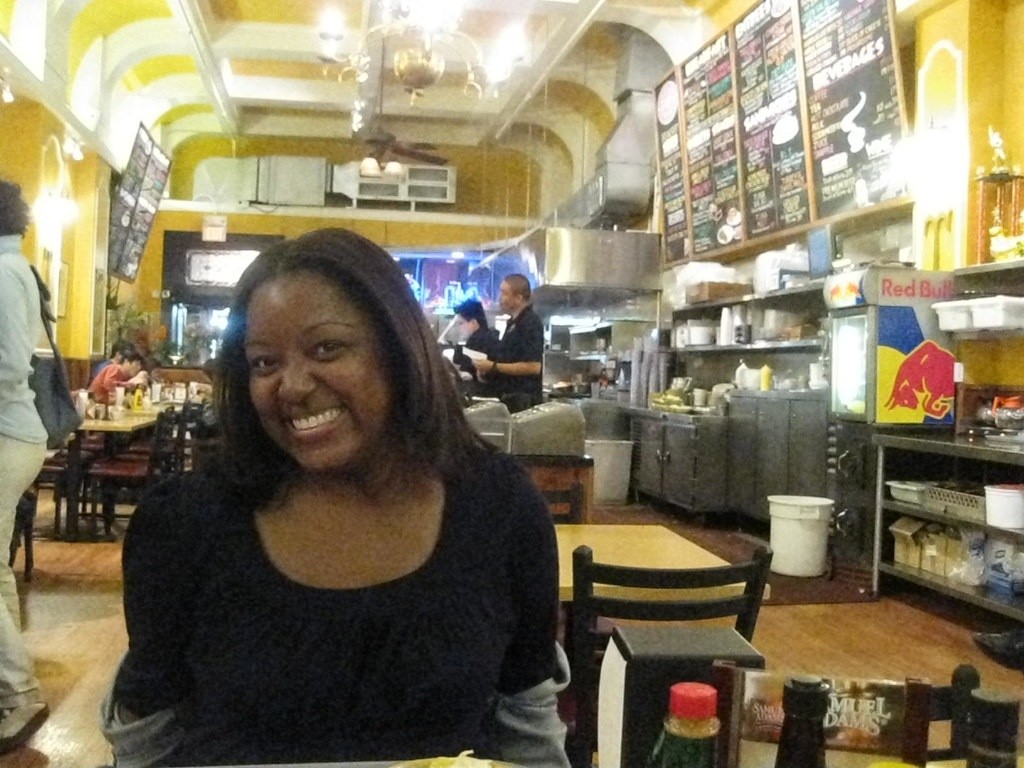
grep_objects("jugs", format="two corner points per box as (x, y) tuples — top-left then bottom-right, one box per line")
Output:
(991, 395), (1024, 430)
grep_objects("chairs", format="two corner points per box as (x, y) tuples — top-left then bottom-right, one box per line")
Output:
(566, 539), (774, 709)
(33, 381), (212, 544)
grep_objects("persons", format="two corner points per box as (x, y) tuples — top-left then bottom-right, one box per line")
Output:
(98, 227), (573, 768)
(454, 300), (501, 377)
(471, 273), (545, 413)
(1, 178), (53, 752)
(89, 339), (147, 406)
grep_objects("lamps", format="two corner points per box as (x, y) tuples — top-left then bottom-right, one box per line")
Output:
(357, 131), (405, 178)
(314, 1), (527, 111)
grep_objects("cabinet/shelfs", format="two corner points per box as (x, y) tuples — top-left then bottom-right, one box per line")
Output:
(625, 409), (725, 514)
(869, 432), (1024, 635)
(666, 275), (829, 355)
(947, 260), (1024, 340)
(728, 391), (828, 525)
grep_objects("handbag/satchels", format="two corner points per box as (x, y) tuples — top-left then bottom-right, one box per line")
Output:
(30, 264), (83, 451)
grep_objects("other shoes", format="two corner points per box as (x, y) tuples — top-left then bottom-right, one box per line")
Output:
(0, 701), (49, 752)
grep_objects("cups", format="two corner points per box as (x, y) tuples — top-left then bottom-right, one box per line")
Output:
(675, 304), (802, 346)
(629, 335), (668, 408)
(691, 388), (708, 405)
(591, 382), (599, 399)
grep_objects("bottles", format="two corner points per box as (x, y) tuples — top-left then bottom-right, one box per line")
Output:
(646, 681), (720, 768)
(775, 673), (830, 768)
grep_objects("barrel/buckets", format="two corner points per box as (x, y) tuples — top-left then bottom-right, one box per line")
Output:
(583, 440), (634, 506)
(766, 494), (835, 577)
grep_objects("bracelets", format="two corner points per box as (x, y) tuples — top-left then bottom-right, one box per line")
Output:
(490, 361), (497, 374)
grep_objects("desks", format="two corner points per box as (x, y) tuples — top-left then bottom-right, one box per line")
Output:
(550, 523), (771, 631)
